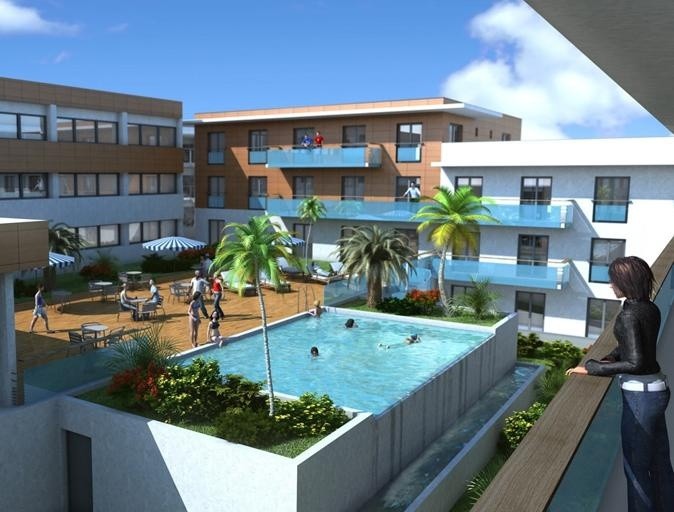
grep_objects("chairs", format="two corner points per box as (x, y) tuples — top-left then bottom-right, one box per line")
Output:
(115, 270), (211, 323)
(220, 256), (345, 299)
(88, 280), (104, 303)
(69, 326), (125, 352)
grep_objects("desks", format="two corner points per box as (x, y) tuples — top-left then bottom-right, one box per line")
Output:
(81, 322), (109, 348)
(94, 282), (112, 301)
(52, 291), (72, 313)
